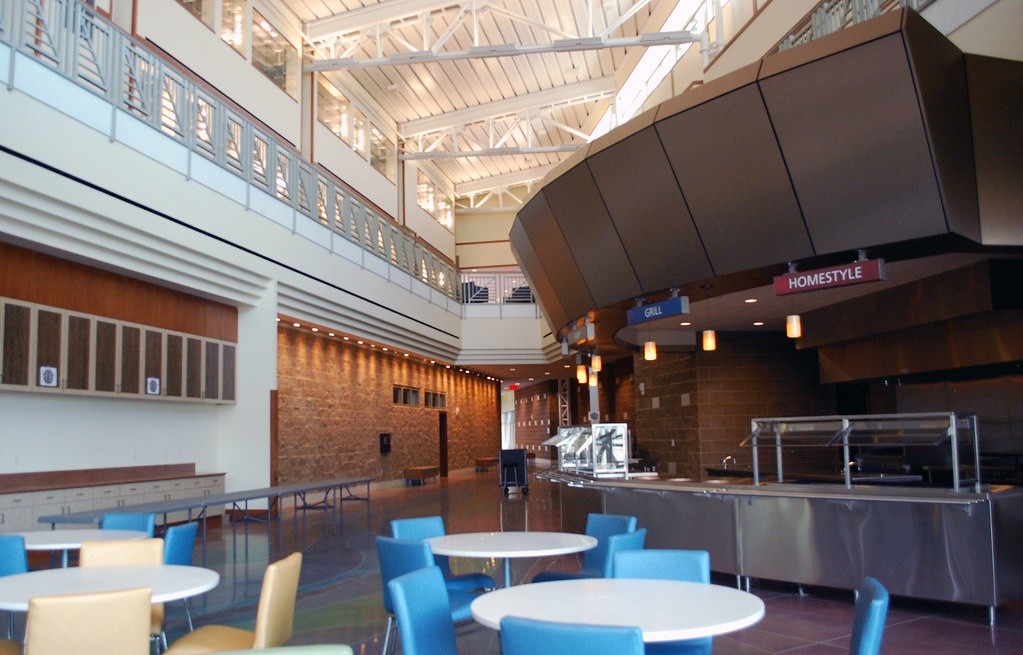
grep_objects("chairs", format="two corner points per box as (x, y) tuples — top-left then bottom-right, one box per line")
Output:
(849, 574), (888, 655)
(0, 511), (713, 655)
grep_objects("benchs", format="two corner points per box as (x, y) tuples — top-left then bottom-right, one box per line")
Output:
(404, 466), (439, 486)
(475, 458), (499, 472)
(527, 453), (535, 466)
(456, 281), (488, 303)
(503, 287), (535, 303)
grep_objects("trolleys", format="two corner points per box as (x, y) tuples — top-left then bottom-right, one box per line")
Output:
(498, 448), (530, 495)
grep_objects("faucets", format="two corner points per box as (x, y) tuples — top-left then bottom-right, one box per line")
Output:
(841, 460), (862, 475)
(721, 455), (736, 469)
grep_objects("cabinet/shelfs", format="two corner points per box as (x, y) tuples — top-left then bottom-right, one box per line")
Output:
(0, 463), (225, 534)
(0, 295), (238, 405)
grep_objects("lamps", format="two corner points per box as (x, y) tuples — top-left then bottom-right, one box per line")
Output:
(785, 295), (801, 338)
(644, 323), (656, 360)
(703, 303), (716, 350)
(576, 345), (603, 386)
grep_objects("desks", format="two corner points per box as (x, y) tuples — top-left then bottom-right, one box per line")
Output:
(38, 477), (376, 569)
(0, 530), (149, 568)
(922, 465), (1021, 485)
(0, 567), (220, 612)
(424, 532), (598, 587)
(471, 578), (765, 642)
